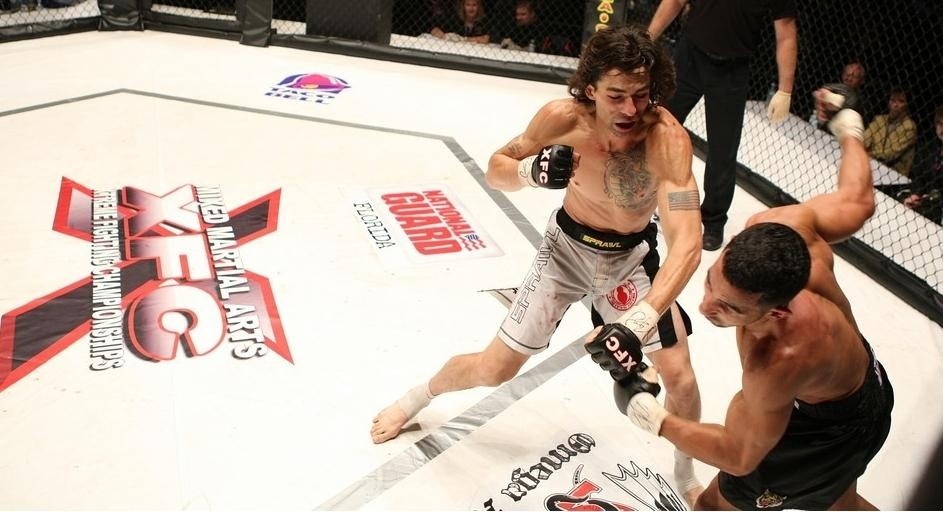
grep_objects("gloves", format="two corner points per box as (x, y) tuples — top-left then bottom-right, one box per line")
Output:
(766, 90), (792, 123)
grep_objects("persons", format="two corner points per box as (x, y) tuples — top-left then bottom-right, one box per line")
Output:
(863, 80), (919, 185)
(891, 105), (942, 223)
(807, 62), (874, 136)
(430, 0), (504, 45)
(612, 78), (897, 510)
(368, 25), (706, 508)
(497, 1), (546, 56)
(644, 0), (800, 254)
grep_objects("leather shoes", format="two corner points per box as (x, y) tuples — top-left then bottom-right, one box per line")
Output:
(703, 229), (723, 251)
